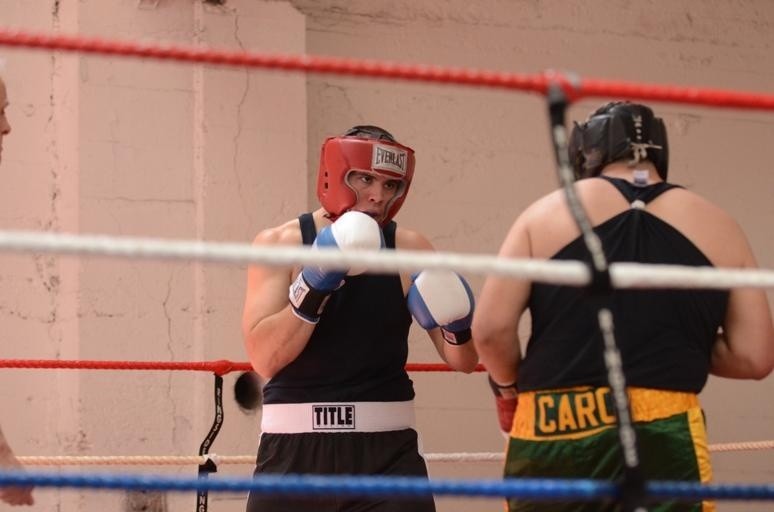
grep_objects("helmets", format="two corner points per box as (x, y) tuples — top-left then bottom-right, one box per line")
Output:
(567, 99), (671, 181)
(315, 124), (417, 229)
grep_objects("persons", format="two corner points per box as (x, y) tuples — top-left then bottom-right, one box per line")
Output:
(240, 124), (481, 509)
(473, 100), (773, 512)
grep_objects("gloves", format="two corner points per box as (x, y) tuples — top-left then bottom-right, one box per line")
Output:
(287, 209), (387, 325)
(487, 375), (518, 434)
(405, 267), (474, 346)
